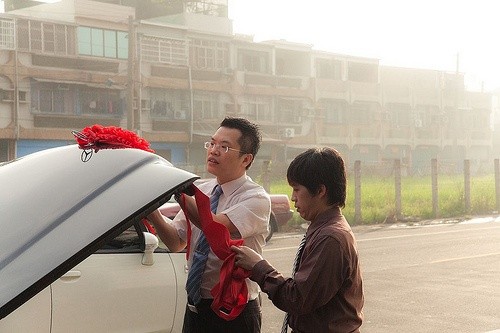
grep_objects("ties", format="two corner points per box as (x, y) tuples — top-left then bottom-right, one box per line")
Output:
(185, 185), (223, 302)
(281, 230), (308, 333)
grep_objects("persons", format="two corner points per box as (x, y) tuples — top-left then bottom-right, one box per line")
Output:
(231, 146), (365, 333)
(149, 115), (272, 333)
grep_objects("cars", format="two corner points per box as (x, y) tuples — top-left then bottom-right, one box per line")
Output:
(0, 214), (261, 333)
(0, 125), (203, 321)
(148, 177), (295, 243)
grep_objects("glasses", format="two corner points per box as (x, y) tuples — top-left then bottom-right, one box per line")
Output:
(204, 141), (246, 153)
